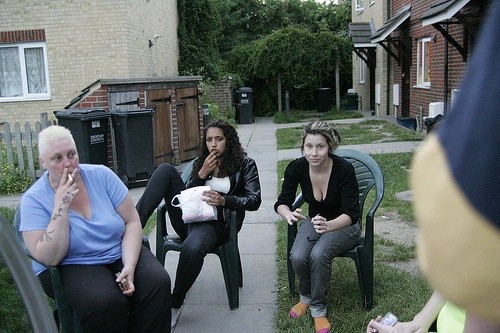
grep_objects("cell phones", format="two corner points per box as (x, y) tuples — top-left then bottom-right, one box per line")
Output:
(372, 312), (398, 332)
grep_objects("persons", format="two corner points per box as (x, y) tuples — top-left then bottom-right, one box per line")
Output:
(136, 118), (261, 329)
(19, 125), (171, 333)
(367, 0), (500, 333)
(274, 121), (362, 333)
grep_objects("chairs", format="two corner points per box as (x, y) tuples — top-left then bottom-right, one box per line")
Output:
(14, 206), (151, 333)
(156, 157), (242, 311)
(288, 149), (384, 310)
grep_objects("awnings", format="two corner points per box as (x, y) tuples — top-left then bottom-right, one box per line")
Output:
(419, 0), (470, 27)
(348, 4), (412, 49)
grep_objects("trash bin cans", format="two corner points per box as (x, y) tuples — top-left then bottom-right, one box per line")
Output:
(110, 110), (154, 188)
(344, 92), (358, 111)
(425, 118), (442, 133)
(314, 88), (330, 112)
(396, 116), (416, 131)
(54, 108), (111, 167)
(236, 87), (256, 125)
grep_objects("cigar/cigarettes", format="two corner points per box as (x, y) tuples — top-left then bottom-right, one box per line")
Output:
(68, 173), (73, 181)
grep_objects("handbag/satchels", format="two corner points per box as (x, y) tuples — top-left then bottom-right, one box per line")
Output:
(171, 187), (218, 224)
